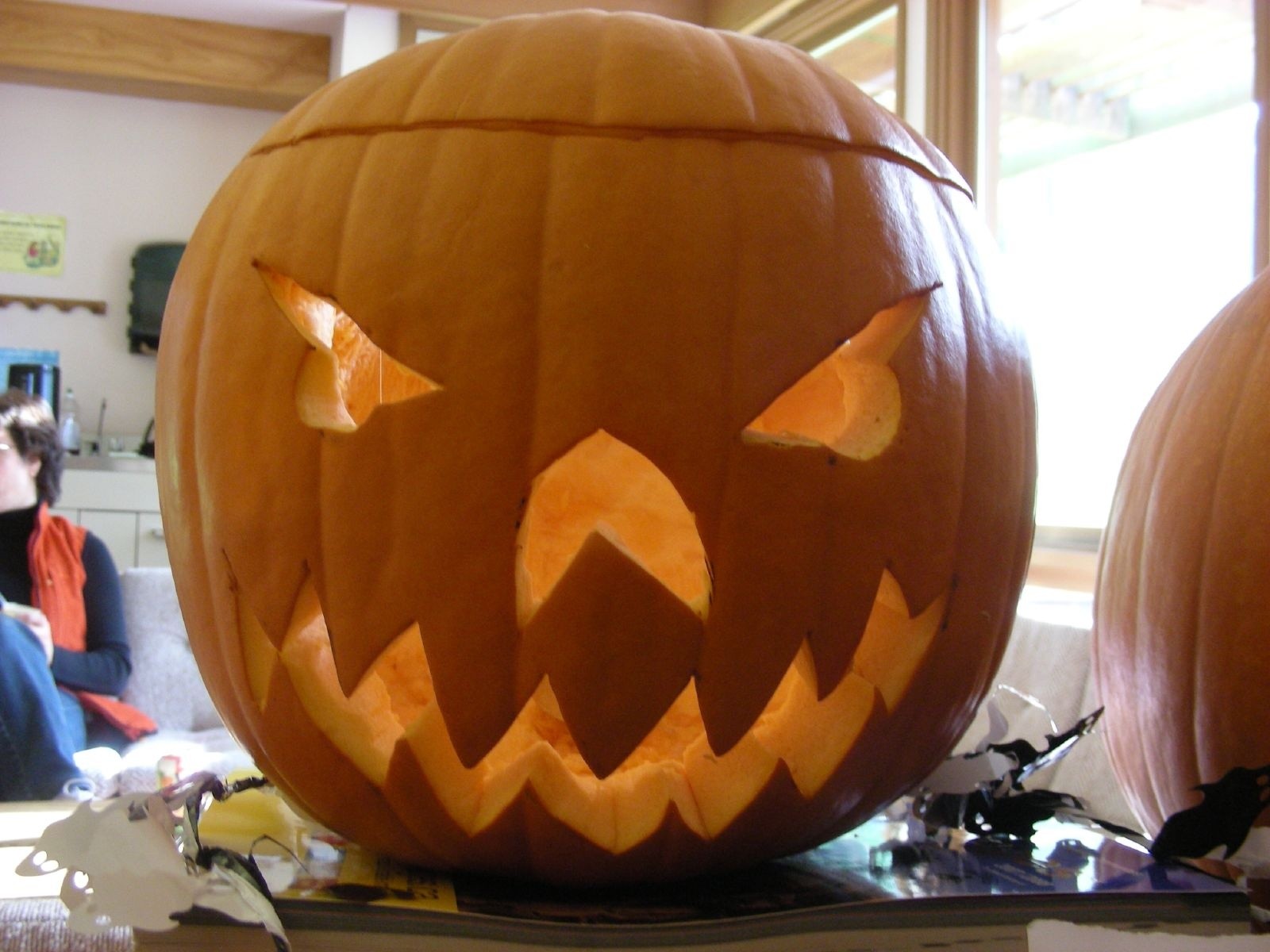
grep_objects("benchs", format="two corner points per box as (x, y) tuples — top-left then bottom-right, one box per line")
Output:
(85, 567), (258, 753)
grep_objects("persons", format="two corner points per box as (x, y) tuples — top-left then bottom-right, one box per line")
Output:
(0, 382), (155, 752)
(0, 615), (90, 803)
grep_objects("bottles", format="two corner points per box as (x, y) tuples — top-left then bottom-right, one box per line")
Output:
(58, 388), (83, 451)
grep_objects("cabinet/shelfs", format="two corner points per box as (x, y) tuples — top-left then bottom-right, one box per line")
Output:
(50, 510), (170, 571)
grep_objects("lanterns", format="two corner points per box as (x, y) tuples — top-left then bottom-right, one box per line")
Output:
(1090, 263), (1270, 894)
(152, 8), (1037, 889)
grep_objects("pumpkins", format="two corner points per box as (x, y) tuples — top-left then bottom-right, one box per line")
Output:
(1095, 263), (1270, 914)
